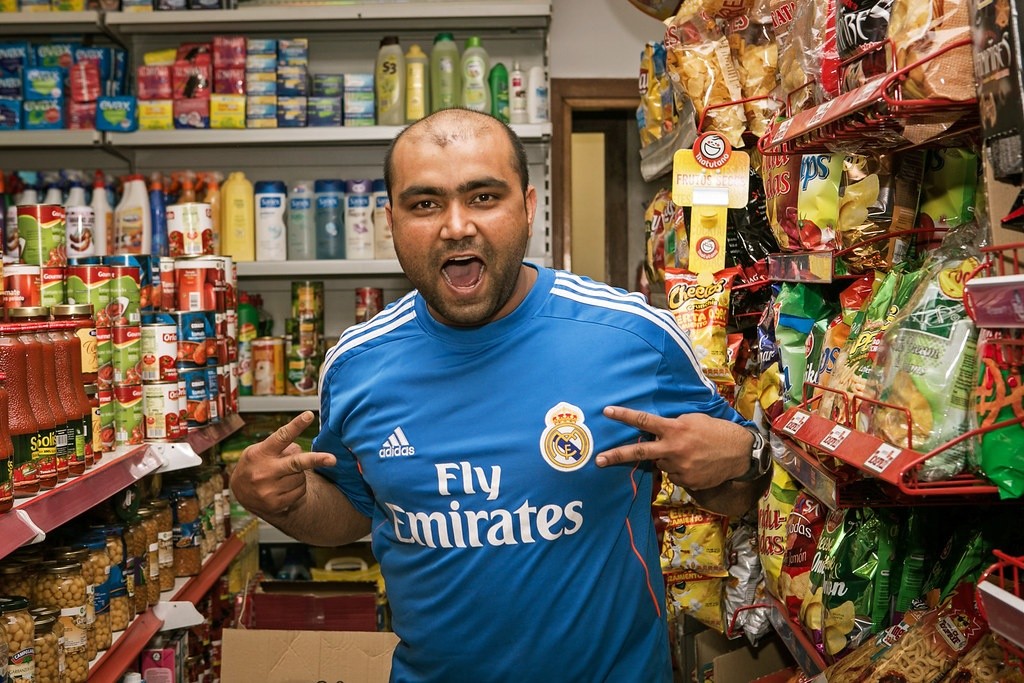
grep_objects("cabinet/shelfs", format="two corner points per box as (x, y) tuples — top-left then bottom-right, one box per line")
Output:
(106, 1), (557, 547)
(143, 413), (250, 630)
(637, 32), (1024, 683)
(12, 444), (165, 683)
(0, 9), (128, 170)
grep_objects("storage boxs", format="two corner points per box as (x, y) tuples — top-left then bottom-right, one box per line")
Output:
(219, 577), (401, 683)
(0, 37), (375, 132)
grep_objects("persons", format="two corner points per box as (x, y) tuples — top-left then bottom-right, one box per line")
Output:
(229, 107), (774, 683)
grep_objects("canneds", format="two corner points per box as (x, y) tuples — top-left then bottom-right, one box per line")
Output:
(251, 279), (385, 398)
(0, 464), (232, 683)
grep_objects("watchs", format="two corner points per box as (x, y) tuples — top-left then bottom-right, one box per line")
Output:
(724, 425), (773, 483)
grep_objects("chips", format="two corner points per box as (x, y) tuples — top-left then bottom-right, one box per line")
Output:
(765, 571), (855, 656)
(666, 38), (779, 118)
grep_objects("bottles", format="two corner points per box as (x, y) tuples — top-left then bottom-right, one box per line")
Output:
(0, 323), (40, 512)
(36, 322), (69, 482)
(65, 321), (93, 468)
(237, 291), (274, 397)
(49, 322), (85, 477)
(20, 323), (58, 489)
